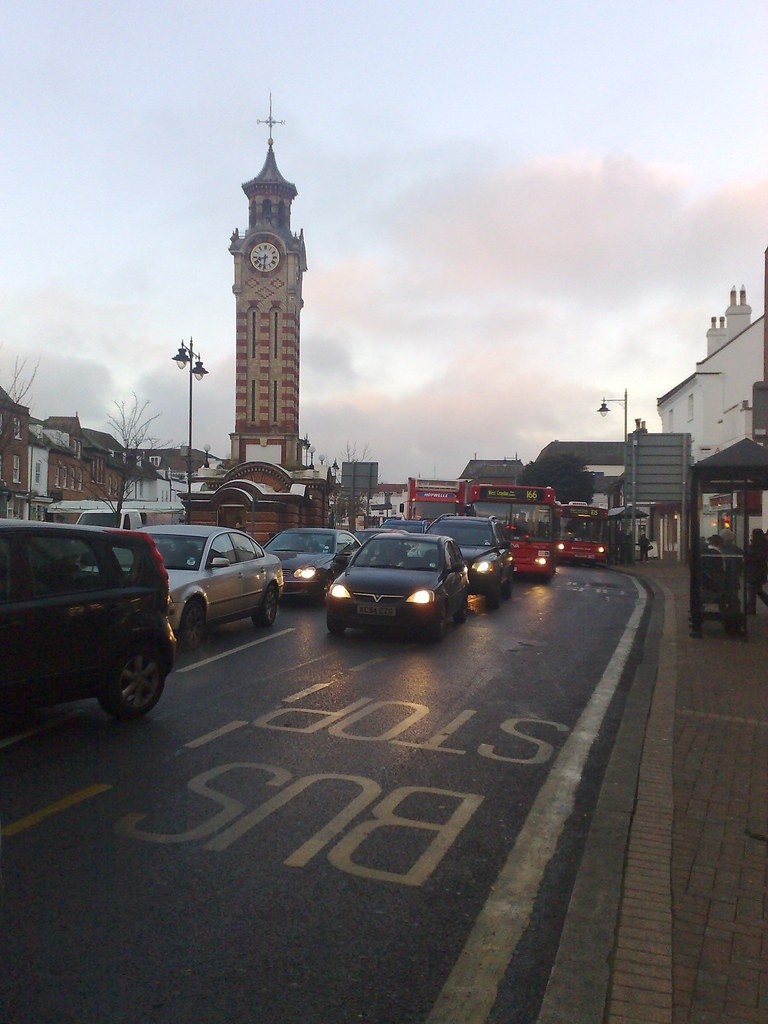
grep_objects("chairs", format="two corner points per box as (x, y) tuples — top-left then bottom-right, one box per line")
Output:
(79, 552), (99, 585)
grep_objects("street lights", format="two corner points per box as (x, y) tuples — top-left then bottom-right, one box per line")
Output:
(383, 489), (394, 517)
(501, 452), (518, 484)
(596, 390), (629, 567)
(170, 336), (210, 527)
(331, 457), (340, 526)
(302, 432), (311, 470)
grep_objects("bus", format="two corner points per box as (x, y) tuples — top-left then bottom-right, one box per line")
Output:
(554, 499), (609, 569)
(462, 482), (562, 580)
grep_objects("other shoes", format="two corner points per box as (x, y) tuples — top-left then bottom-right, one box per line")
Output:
(747, 608), (756, 614)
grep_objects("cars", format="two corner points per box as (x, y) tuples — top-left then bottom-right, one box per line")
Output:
(261, 528), (369, 606)
(324, 532), (471, 641)
(81, 523), (284, 652)
(351, 526), (410, 550)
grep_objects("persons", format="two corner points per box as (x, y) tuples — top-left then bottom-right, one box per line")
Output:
(616, 525), (633, 564)
(744, 528), (768, 614)
(516, 513), (529, 535)
(699, 528), (744, 592)
(639, 534), (650, 564)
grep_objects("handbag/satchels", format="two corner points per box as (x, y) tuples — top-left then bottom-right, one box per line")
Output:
(648, 546), (653, 551)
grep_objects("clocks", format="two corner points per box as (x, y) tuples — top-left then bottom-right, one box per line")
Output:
(250, 242), (280, 272)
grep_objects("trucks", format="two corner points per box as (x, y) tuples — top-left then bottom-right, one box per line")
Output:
(399, 475), (477, 523)
(75, 508), (144, 532)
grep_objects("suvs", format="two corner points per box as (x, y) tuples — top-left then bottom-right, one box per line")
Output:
(408, 512), (515, 609)
(378, 517), (431, 545)
(0, 517), (178, 722)
